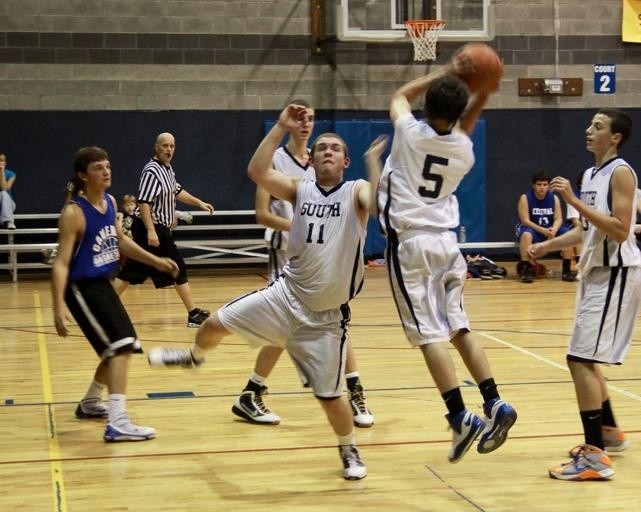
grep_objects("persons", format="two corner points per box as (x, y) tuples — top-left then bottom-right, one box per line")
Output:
(148, 49), (517, 481)
(567, 178), (586, 275)
(0, 154), (17, 229)
(514, 168), (577, 283)
(51, 132), (214, 442)
(525, 108), (641, 482)
(634, 187), (641, 243)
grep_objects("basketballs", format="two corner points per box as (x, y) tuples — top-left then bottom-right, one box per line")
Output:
(456, 44), (500, 95)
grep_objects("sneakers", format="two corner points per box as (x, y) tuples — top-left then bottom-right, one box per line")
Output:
(447, 398), (517, 464)
(549, 426), (628, 480)
(347, 377), (374, 427)
(232, 390), (280, 424)
(76, 398), (155, 443)
(519, 261), (532, 282)
(187, 309), (210, 327)
(149, 348), (202, 370)
(561, 266), (574, 280)
(339, 444), (367, 479)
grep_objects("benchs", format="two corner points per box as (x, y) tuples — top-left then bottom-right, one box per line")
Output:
(0, 210), (269, 282)
(458, 241), (520, 249)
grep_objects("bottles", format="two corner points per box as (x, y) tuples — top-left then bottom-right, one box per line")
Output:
(459, 224), (466, 243)
